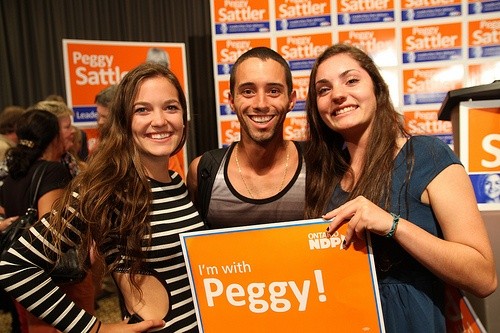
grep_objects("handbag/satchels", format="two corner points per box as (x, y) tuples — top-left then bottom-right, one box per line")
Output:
(0, 160), (89, 289)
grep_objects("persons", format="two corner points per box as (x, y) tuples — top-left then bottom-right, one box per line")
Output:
(147, 48), (170, 67)
(0, 63), (206, 333)
(187, 47), (306, 228)
(0, 85), (120, 333)
(306, 44), (498, 333)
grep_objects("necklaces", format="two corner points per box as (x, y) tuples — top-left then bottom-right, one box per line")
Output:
(235, 140), (289, 199)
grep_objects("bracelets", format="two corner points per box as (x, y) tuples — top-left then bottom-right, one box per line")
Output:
(384, 212), (400, 238)
